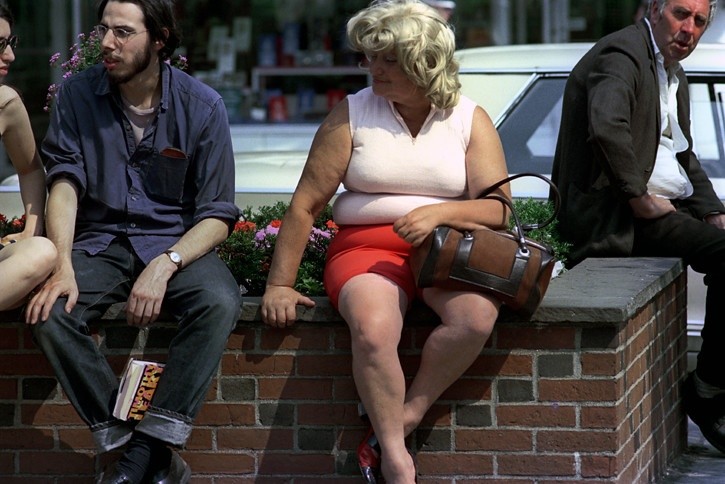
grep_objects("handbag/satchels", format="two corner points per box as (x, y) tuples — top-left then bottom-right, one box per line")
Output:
(418, 172), (562, 323)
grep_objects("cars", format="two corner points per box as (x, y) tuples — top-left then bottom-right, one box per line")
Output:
(446, 40), (725, 377)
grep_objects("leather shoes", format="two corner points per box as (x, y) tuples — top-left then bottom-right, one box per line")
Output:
(147, 447), (191, 484)
(97, 458), (134, 484)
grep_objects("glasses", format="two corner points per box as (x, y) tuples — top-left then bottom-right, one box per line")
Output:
(0, 36), (17, 53)
(96, 25), (157, 44)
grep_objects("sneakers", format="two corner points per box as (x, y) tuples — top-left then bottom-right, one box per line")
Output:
(682, 370), (725, 453)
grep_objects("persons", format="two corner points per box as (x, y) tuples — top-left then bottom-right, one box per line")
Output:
(547, 0), (724, 456)
(0, 9), (59, 314)
(25, 2), (241, 484)
(260, 1), (514, 484)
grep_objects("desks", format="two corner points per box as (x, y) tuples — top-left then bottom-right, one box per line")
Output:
(248, 67), (376, 107)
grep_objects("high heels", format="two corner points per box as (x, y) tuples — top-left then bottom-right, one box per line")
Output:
(356, 403), (382, 484)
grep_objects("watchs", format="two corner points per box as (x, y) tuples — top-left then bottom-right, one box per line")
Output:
(163, 248), (185, 270)
